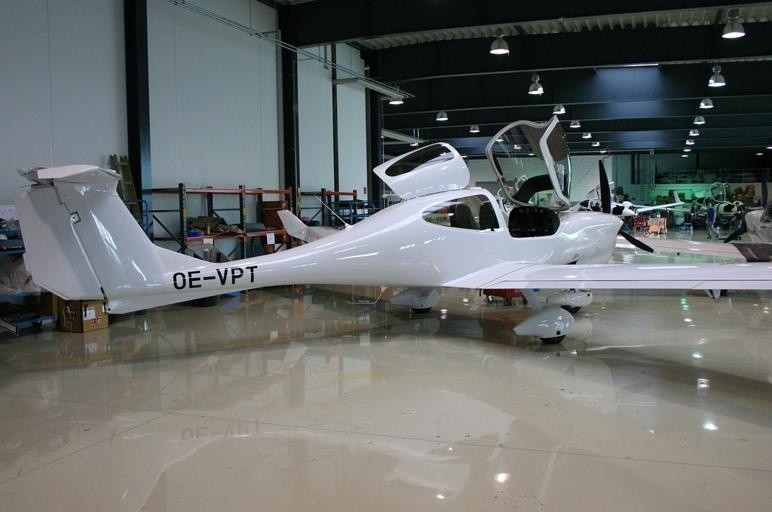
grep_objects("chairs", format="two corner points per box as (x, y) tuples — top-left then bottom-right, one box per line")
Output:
(447, 202), (499, 231)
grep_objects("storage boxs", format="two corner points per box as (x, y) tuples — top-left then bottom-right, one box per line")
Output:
(57, 298), (110, 333)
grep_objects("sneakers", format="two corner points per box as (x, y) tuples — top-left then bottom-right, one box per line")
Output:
(706, 236), (712, 239)
(716, 230), (719, 238)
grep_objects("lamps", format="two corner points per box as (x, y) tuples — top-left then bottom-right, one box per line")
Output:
(688, 12), (745, 137)
(387, 94), (480, 134)
(488, 33), (592, 139)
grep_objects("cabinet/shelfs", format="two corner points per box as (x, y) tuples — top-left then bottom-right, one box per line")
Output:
(144, 183), (356, 263)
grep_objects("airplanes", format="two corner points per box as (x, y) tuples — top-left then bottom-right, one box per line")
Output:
(11, 112), (684, 345)
(690, 182), (764, 226)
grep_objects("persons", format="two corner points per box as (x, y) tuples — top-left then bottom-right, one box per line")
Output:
(592, 203), (600, 211)
(620, 194), (635, 232)
(732, 202), (743, 240)
(706, 202), (719, 239)
